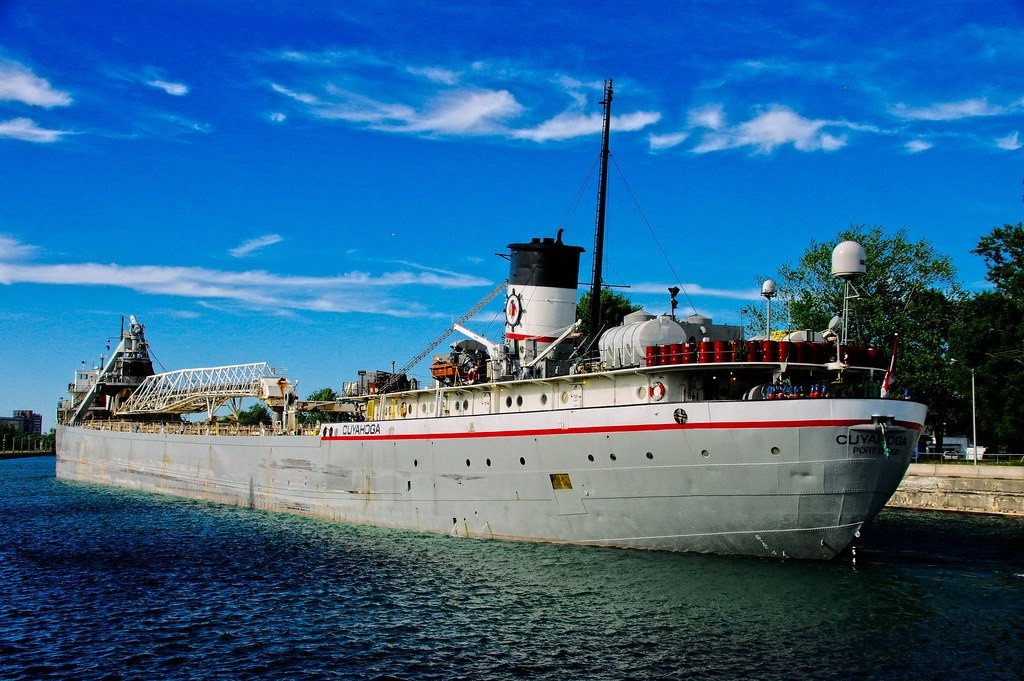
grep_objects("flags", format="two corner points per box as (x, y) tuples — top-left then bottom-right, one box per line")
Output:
(880, 335), (899, 398)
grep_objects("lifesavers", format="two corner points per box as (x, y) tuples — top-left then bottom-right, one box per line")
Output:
(467, 367), (477, 385)
(648, 381), (665, 401)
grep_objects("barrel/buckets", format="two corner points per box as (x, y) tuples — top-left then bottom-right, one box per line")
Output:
(645, 340), (887, 368)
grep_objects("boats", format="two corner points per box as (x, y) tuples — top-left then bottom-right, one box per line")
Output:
(50, 78), (930, 572)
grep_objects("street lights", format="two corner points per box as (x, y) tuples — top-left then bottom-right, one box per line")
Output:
(951, 358), (977, 467)
